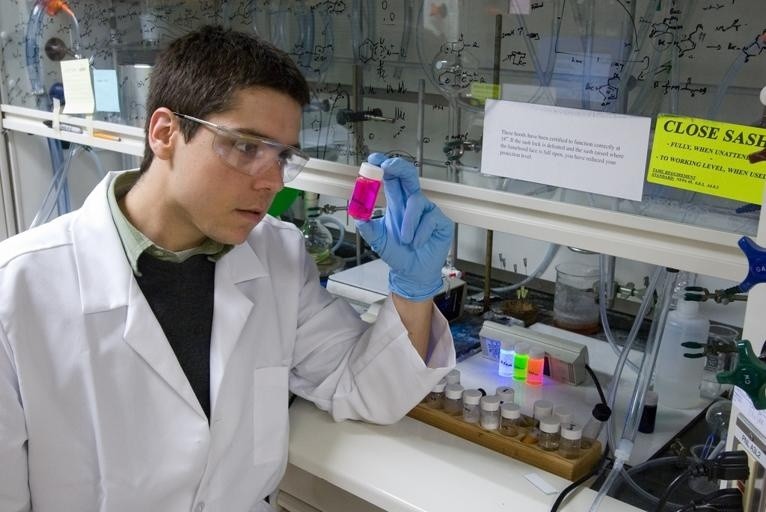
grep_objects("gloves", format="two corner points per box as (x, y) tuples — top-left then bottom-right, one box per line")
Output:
(355, 152), (454, 301)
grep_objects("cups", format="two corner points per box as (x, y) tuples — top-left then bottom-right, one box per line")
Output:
(701, 324), (739, 400)
(553, 263), (601, 335)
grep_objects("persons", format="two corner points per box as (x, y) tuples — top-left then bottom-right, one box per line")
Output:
(0, 25), (454, 511)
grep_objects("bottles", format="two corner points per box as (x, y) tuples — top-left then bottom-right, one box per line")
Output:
(538, 417), (559, 451)
(532, 400), (553, 434)
(512, 342), (530, 382)
(426, 378), (447, 409)
(582, 403), (611, 449)
(636, 391), (658, 434)
(499, 402), (520, 436)
(461, 389), (482, 424)
(479, 395), (500, 431)
(501, 298), (542, 327)
(654, 299), (710, 410)
(445, 369), (461, 385)
(496, 387), (515, 405)
(559, 421), (583, 460)
(498, 338), (516, 377)
(348, 162), (383, 222)
(553, 406), (575, 424)
(443, 385), (464, 415)
(526, 347), (545, 387)
(300, 200), (333, 265)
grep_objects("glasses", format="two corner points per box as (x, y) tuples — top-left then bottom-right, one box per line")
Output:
(172, 111), (309, 182)
